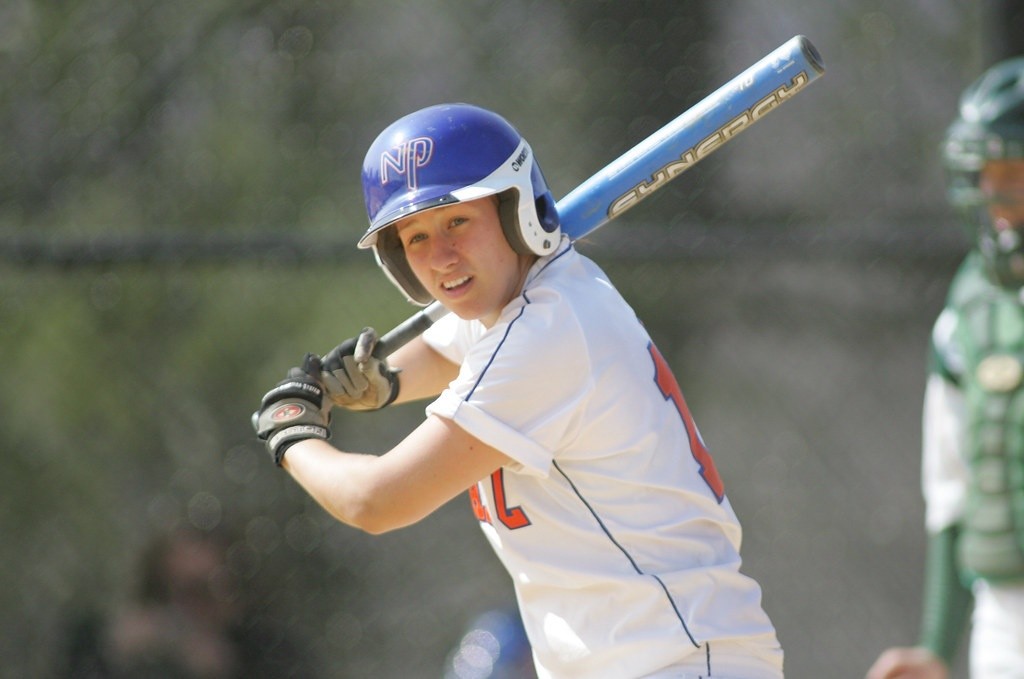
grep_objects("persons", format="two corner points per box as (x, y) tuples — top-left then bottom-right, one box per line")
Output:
(81, 521), (250, 679)
(446, 605), (537, 679)
(867, 56), (1023, 677)
(252, 103), (786, 678)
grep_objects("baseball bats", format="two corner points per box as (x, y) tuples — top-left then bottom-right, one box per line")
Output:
(250, 32), (830, 436)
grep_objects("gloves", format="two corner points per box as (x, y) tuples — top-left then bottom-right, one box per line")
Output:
(321, 327), (402, 411)
(250, 352), (331, 467)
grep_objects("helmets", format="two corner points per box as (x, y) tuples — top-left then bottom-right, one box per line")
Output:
(357, 103), (561, 307)
(936, 57), (1024, 290)
